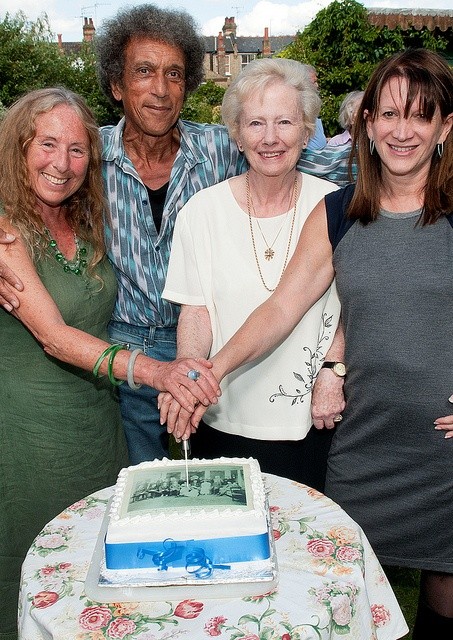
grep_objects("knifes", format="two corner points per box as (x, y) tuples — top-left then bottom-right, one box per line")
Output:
(181, 438), (189, 488)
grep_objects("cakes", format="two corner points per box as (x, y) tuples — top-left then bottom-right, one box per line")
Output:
(96, 455), (276, 585)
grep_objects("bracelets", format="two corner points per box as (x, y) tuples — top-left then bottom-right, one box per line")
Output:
(108, 343), (127, 386)
(127, 348), (144, 390)
(93, 343), (120, 379)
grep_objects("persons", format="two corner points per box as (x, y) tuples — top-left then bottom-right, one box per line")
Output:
(301, 64), (327, 150)
(156, 58), (347, 495)
(0, 4), (357, 467)
(327, 90), (366, 146)
(0, 88), (221, 640)
(156, 48), (453, 640)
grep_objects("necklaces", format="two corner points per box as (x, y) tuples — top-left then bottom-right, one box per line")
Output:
(245, 169), (298, 291)
(248, 178), (295, 261)
(39, 221), (88, 276)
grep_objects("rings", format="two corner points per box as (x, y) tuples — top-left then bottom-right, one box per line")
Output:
(334, 415), (343, 422)
(188, 369), (199, 383)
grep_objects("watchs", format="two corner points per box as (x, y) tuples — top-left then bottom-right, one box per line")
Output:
(322, 362), (346, 377)
